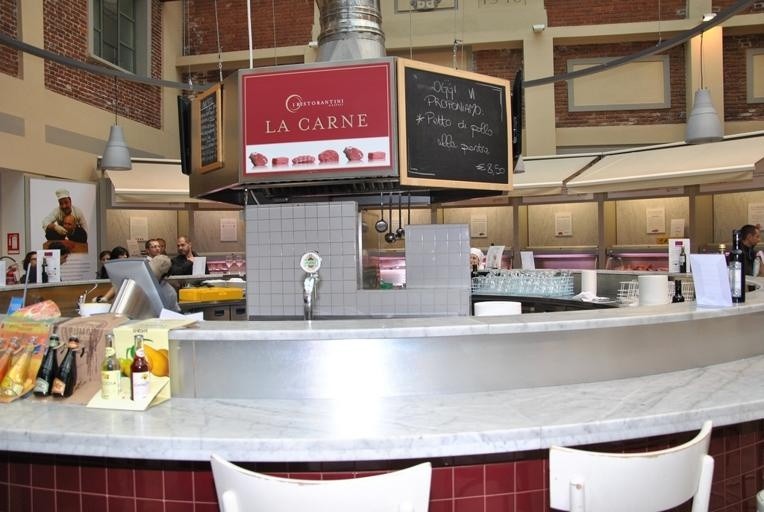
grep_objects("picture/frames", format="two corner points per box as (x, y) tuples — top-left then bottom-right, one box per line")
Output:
(237, 56), (399, 185)
(23, 174), (101, 281)
(506, 0), (528, 8)
(478, 0), (500, 8)
(567, 55), (671, 112)
(394, 0), (458, 14)
(746, 48), (764, 103)
(485, 245), (505, 268)
(520, 252), (535, 269)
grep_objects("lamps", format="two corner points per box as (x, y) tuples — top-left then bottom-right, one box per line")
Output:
(101, 47), (133, 171)
(684, 30), (724, 144)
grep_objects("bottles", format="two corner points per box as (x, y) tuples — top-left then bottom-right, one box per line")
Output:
(730, 229), (745, 302)
(672, 279), (684, 303)
(130, 335), (150, 402)
(99, 336), (121, 399)
(718, 243), (729, 279)
(0, 336), (18, 382)
(0, 337), (37, 398)
(679, 245), (686, 274)
(32, 334), (59, 397)
(50, 335), (79, 398)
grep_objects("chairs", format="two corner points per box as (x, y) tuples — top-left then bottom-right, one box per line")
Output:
(549, 420), (715, 512)
(206, 453), (432, 511)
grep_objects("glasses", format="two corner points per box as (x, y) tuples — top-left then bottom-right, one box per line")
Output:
(101, 257), (111, 261)
(150, 245), (160, 248)
(30, 257), (37, 261)
(161, 245), (165, 247)
(177, 243), (186, 247)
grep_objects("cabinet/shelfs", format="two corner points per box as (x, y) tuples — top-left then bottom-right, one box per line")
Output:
(0, 277), (764, 512)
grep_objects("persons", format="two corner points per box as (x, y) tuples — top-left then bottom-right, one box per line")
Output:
(154, 238), (167, 256)
(110, 246), (130, 260)
(144, 239), (159, 263)
(469, 247), (484, 272)
(48, 242), (70, 265)
(734, 225), (763, 276)
(42, 188), (85, 233)
(45, 214), (86, 241)
(17, 252), (37, 284)
(99, 250), (110, 277)
(168, 237), (209, 275)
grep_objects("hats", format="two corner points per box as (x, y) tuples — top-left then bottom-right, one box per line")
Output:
(470, 247), (484, 265)
(55, 187), (71, 200)
